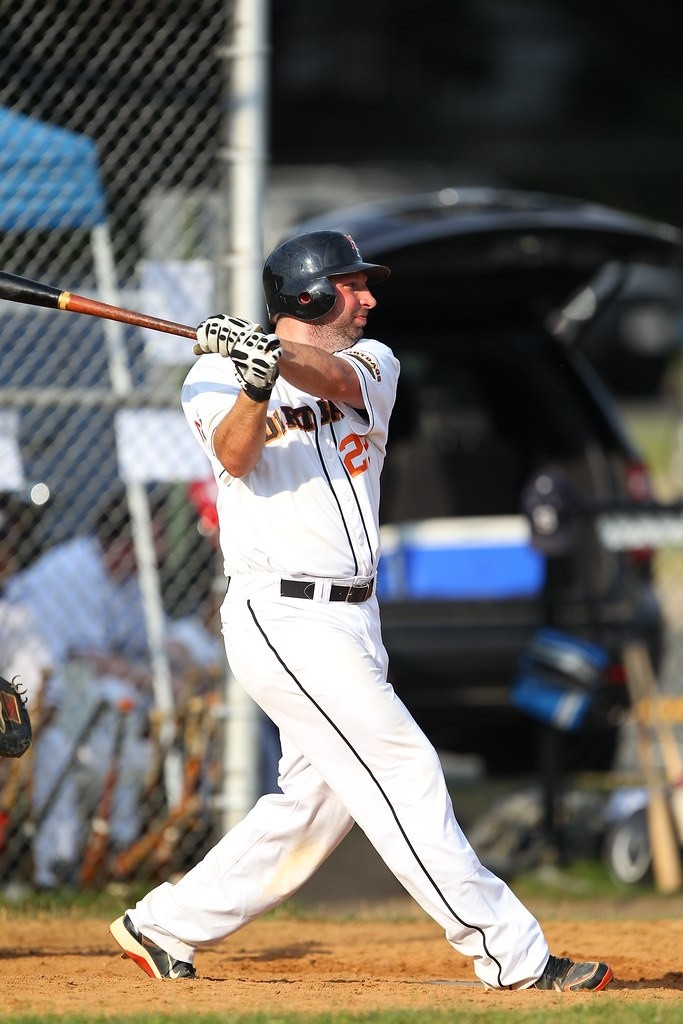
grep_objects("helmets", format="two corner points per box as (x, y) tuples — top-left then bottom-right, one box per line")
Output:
(263, 230), (390, 324)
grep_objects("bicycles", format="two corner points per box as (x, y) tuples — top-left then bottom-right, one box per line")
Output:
(525, 455), (683, 871)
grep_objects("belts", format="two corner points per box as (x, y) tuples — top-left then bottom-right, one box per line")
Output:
(224, 576), (376, 604)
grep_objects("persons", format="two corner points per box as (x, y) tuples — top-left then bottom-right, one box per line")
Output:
(108, 229), (620, 992)
(11, 477), (213, 894)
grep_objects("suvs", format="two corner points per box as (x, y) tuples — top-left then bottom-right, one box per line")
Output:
(0, 185), (683, 833)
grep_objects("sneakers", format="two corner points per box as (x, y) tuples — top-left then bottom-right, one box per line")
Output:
(526, 954), (614, 992)
(110, 912), (198, 980)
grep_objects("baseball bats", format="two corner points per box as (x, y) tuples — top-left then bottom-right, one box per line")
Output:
(622, 640), (683, 895)
(0, 270), (197, 339)
(0, 693), (222, 889)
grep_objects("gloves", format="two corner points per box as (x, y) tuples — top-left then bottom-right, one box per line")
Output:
(229, 323), (283, 403)
(192, 313), (254, 357)
